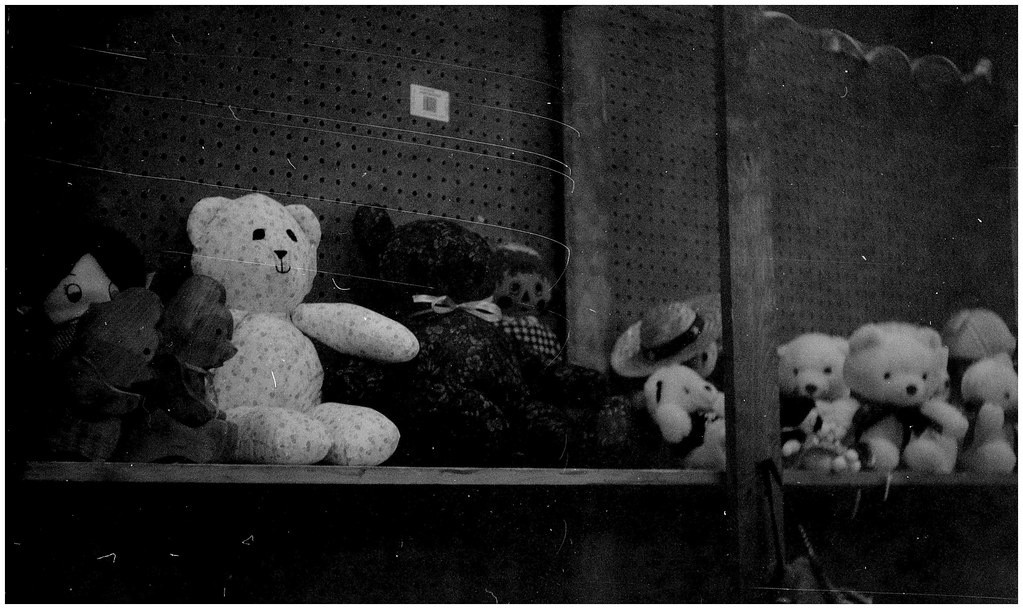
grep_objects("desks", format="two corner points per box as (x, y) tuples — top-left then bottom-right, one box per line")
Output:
(4, 462), (1017, 604)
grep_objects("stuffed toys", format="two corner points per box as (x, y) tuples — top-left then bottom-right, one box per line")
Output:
(36, 285), (166, 462)
(154, 273), (276, 466)
(832, 320), (970, 479)
(186, 193), (421, 469)
(332, 201), (571, 467)
(608, 295), (825, 458)
(491, 240), (571, 376)
(775, 331), (865, 478)
(943, 309), (1019, 476)
(642, 364), (729, 472)
(11, 240), (120, 374)
